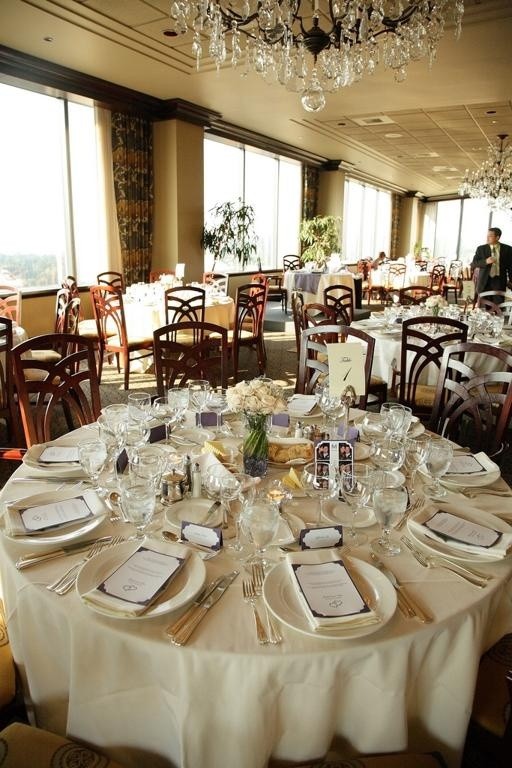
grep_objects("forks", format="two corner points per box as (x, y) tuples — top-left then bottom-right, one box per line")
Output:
(241, 562), (285, 646)
(46, 535), (125, 595)
(396, 534), (493, 588)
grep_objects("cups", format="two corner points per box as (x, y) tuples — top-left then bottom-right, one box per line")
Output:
(103, 403), (129, 421)
(391, 405), (413, 434)
(127, 391), (152, 424)
(254, 476), (294, 516)
(381, 403), (405, 433)
(361, 413), (395, 444)
(116, 416), (151, 456)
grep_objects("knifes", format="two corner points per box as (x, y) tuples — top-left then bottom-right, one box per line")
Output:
(163, 567), (243, 648)
(199, 501), (221, 525)
(371, 553), (431, 627)
(278, 505), (300, 545)
(11, 534), (109, 571)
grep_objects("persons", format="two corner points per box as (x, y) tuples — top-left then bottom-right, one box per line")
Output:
(472, 228), (512, 313)
(316, 444), (353, 491)
(370, 251), (386, 270)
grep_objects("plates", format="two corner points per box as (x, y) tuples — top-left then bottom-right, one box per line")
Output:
(169, 428), (216, 445)
(261, 557), (400, 641)
(372, 470), (406, 489)
(267, 436), (316, 470)
(0, 439), (100, 548)
(74, 540), (209, 621)
(165, 497), (224, 529)
(238, 511), (307, 552)
(408, 449), (512, 566)
(321, 501), (376, 528)
(189, 443), (244, 460)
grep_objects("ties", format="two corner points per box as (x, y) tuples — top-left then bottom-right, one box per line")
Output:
(489, 248), (497, 279)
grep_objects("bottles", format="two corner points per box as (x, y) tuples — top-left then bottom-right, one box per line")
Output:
(183, 455), (194, 491)
(191, 463), (202, 499)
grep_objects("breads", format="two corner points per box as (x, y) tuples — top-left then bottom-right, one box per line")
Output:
(267, 444), (313, 464)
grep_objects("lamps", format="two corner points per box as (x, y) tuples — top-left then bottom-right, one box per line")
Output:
(171, 0), (467, 114)
(458, 133), (512, 210)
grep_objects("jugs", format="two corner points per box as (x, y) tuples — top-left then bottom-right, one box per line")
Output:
(160, 468), (189, 507)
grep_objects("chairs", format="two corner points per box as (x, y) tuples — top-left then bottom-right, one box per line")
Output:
(153, 323), (227, 400)
(277, 251), (512, 343)
(390, 316), (469, 415)
(295, 325), (376, 409)
(423, 342), (512, 465)
(3, 257), (277, 391)
(9, 332), (101, 448)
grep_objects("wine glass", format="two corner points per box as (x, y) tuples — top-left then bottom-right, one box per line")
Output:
(369, 482), (407, 557)
(423, 439), (451, 497)
(152, 397), (179, 448)
(220, 472), (256, 556)
(384, 301), (506, 341)
(120, 473), (158, 540)
(302, 462), (342, 529)
(340, 463), (375, 548)
(164, 377), (354, 441)
(368, 438), (406, 489)
(129, 446), (165, 514)
(204, 462), (246, 540)
(97, 413), (127, 482)
(240, 498), (279, 575)
(399, 432), (433, 495)
(125, 273), (224, 305)
